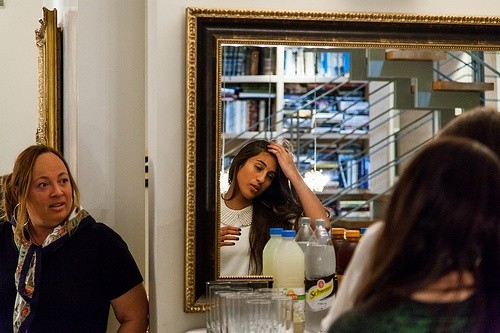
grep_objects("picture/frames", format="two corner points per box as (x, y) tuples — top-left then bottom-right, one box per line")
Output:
(31, 7), (66, 154)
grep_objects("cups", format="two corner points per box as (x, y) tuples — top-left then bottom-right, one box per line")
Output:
(206, 281), (248, 333)
(248, 281), (294, 333)
(228, 281), (271, 333)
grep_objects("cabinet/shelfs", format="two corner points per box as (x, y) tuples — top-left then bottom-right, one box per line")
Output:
(221, 45), (369, 196)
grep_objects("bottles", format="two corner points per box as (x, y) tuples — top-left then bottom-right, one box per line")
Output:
(361, 228), (367, 238)
(294, 217), (314, 253)
(304, 219), (336, 333)
(262, 228), (283, 275)
(335, 230), (360, 289)
(272, 230), (306, 333)
(331, 227), (345, 260)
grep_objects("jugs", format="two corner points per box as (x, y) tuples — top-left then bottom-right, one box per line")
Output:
(339, 159), (362, 190)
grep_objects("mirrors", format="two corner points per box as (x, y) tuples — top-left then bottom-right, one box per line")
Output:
(182, 7), (500, 313)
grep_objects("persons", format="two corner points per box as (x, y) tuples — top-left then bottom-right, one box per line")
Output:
(0, 145), (149, 333)
(219, 140), (331, 278)
(324, 105), (500, 333)
